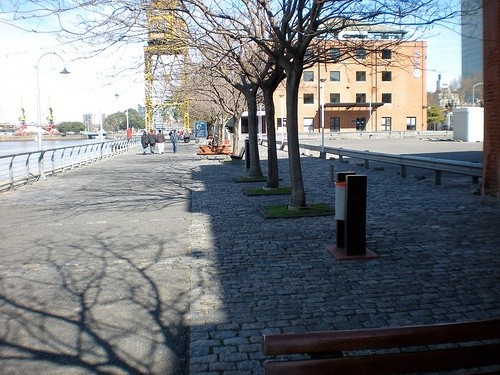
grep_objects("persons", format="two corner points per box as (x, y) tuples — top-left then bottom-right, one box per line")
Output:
(171, 130), (184, 153)
(141, 130), (165, 154)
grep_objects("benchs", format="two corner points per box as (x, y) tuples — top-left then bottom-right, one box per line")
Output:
(263, 318), (500, 375)
(196, 145), (232, 160)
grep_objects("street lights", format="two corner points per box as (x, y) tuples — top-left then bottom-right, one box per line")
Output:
(33, 51), (72, 181)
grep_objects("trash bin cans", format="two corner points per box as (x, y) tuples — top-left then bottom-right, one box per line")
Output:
(245, 138), (250, 172)
(335, 180), (346, 220)
(184, 134), (190, 143)
